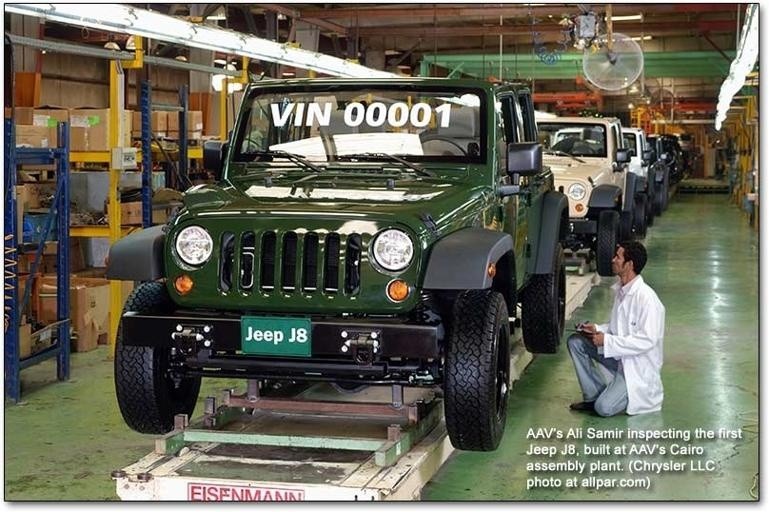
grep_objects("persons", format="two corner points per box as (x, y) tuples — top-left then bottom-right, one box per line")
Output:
(567, 239), (668, 417)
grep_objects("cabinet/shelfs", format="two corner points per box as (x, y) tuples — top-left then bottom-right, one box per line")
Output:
(5, 118), (71, 401)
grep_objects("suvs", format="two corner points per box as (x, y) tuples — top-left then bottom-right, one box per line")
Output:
(105, 79), (570, 453)
(533, 117), (686, 278)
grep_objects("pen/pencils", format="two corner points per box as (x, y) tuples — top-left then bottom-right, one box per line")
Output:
(580, 321), (590, 329)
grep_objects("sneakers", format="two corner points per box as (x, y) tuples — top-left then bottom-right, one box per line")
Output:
(570, 400), (594, 412)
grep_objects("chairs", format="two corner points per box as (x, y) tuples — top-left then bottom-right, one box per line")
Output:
(296, 105), (510, 175)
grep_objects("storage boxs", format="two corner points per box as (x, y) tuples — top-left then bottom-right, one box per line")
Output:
(4, 104), (203, 359)
